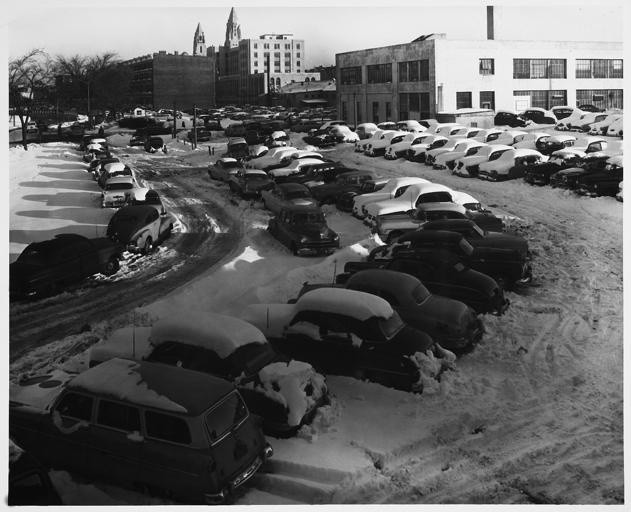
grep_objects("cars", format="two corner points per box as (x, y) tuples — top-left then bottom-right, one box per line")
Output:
(14, 233), (120, 300)
(123, 187), (169, 219)
(297, 268), (485, 356)
(9, 438), (64, 506)
(241, 287), (445, 394)
(78, 104), (623, 260)
(342, 250), (510, 317)
(266, 205), (340, 258)
(106, 204), (176, 253)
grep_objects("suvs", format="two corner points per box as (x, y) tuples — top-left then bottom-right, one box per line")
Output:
(42, 357), (275, 505)
(26, 121), (38, 133)
(143, 312), (330, 439)
